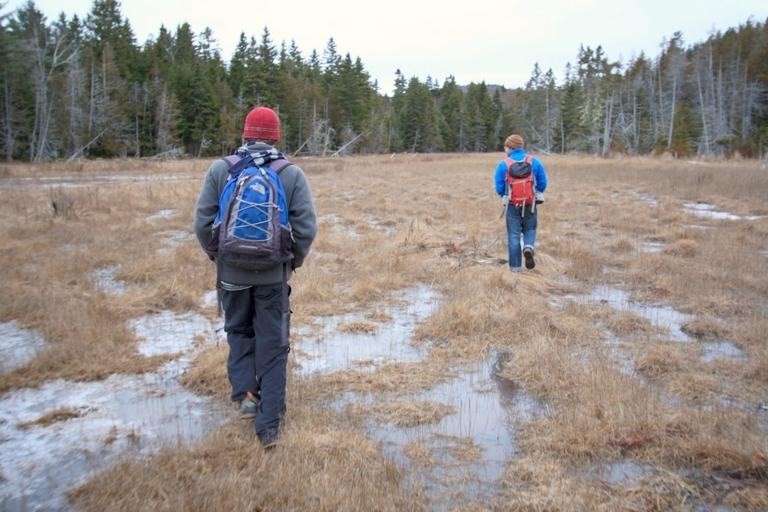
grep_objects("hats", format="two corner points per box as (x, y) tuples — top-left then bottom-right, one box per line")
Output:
(505, 134), (525, 150)
(242, 107), (281, 140)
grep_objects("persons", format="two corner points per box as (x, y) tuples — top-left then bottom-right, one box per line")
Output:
(193, 105), (318, 456)
(496, 134), (549, 275)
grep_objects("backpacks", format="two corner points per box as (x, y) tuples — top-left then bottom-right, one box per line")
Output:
(504, 154), (538, 205)
(207, 156), (295, 268)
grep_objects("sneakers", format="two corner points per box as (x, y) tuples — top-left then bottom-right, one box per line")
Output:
(239, 396), (259, 419)
(524, 247), (535, 269)
(261, 426), (283, 450)
